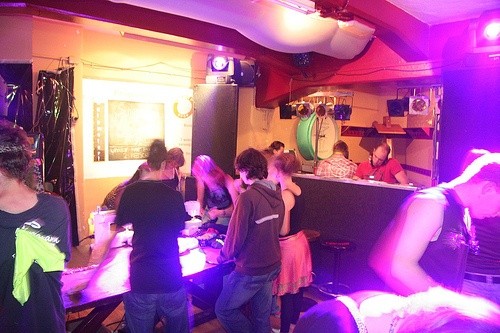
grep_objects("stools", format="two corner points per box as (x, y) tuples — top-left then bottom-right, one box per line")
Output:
(318, 238), (356, 297)
(299, 229), (320, 312)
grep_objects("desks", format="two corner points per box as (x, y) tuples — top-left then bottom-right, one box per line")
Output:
(60, 238), (235, 333)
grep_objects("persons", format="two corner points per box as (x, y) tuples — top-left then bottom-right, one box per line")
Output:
(0, 121), (500, 333)
(352, 143), (409, 186)
(215, 147), (285, 333)
(315, 140), (357, 179)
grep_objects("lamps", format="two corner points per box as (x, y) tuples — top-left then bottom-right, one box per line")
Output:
(205, 54), (257, 88)
(280, 104), (298, 119)
(387, 97), (410, 117)
(315, 102), (334, 118)
(470, 9), (500, 59)
(334, 104), (352, 121)
(409, 94), (431, 115)
(295, 102), (315, 118)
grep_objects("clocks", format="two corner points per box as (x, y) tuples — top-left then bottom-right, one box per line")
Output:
(297, 113), (340, 161)
